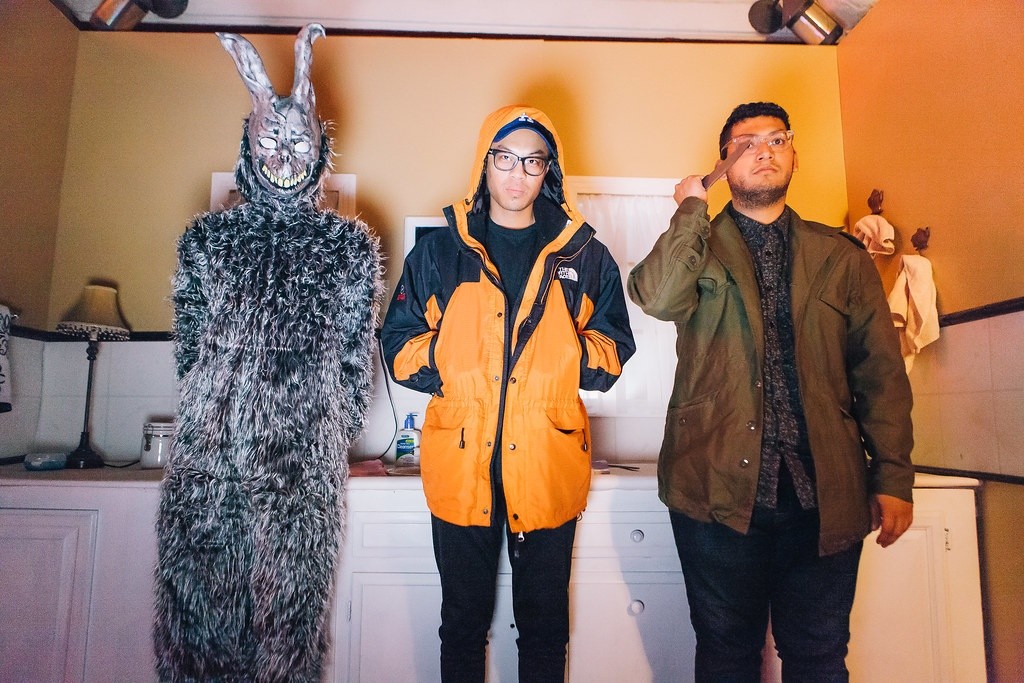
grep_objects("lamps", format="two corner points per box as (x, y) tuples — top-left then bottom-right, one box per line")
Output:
(56, 284), (130, 469)
(91, 0), (188, 31)
(749, 0), (843, 46)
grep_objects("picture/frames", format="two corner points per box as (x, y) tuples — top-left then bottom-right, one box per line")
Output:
(210, 172), (357, 220)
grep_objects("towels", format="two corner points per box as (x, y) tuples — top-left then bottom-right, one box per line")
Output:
(887, 253), (941, 376)
(852, 213), (897, 260)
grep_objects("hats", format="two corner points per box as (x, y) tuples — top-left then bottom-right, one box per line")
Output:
(494, 117), (557, 158)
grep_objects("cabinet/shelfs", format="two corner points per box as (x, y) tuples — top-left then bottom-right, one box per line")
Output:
(0, 462), (989, 683)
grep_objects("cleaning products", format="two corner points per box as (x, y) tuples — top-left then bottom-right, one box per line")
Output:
(393, 411), (423, 466)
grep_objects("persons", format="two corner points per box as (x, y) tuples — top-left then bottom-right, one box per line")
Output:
(149, 98), (387, 683)
(628, 102), (914, 683)
(381, 105), (636, 683)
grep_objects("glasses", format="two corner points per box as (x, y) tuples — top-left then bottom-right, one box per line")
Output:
(487, 149), (553, 176)
(721, 130), (794, 154)
